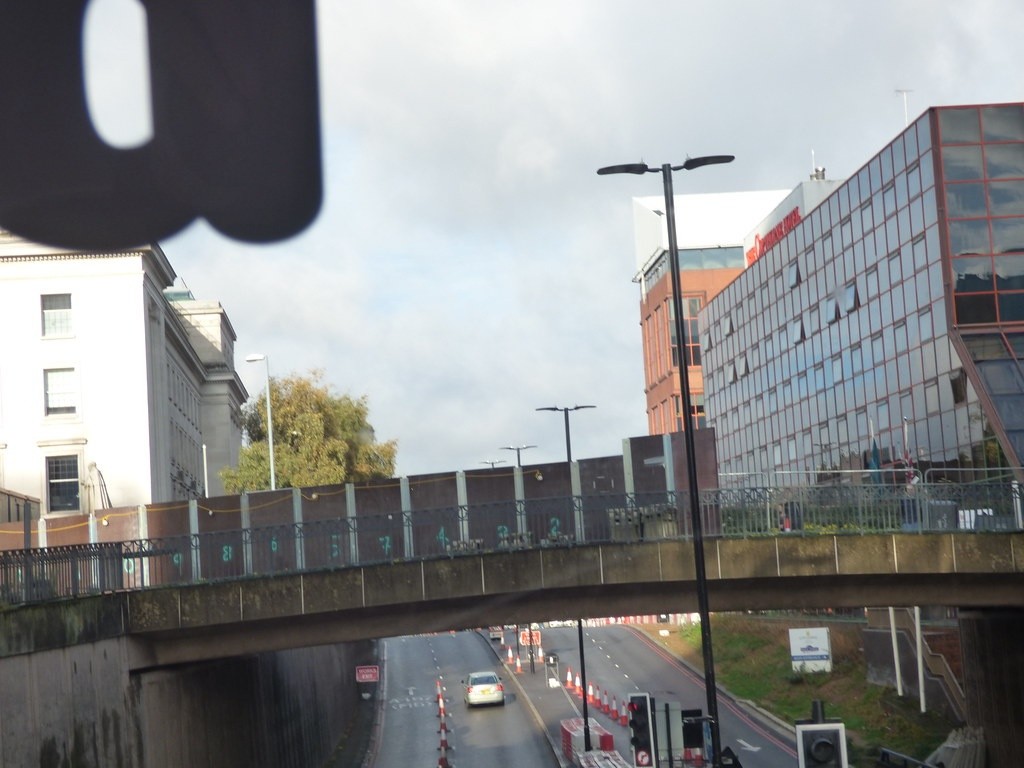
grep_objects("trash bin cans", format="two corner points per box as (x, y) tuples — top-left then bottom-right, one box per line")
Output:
(782, 500), (802, 531)
(605, 503), (680, 541)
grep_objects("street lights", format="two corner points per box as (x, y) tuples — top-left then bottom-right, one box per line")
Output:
(244, 353), (276, 490)
(597, 154), (737, 767)
(478, 461), (507, 468)
(536, 405), (597, 461)
(500, 446), (537, 466)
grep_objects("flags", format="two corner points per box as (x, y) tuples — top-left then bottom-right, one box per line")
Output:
(902, 422), (915, 484)
(868, 441), (882, 483)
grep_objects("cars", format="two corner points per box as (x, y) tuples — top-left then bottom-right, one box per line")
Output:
(461, 671), (505, 708)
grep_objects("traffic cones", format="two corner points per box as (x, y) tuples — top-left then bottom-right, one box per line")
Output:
(683, 748), (706, 768)
(436, 710), (451, 733)
(608, 695), (619, 719)
(434, 681), (445, 702)
(438, 743), (453, 768)
(593, 685), (602, 708)
(505, 646), (515, 664)
(514, 655), (524, 674)
(499, 636), (507, 650)
(600, 690), (611, 714)
(587, 682), (595, 704)
(565, 667), (575, 689)
(572, 672), (582, 694)
(437, 724), (452, 750)
(436, 694), (449, 718)
(618, 700), (628, 726)
(538, 646), (545, 662)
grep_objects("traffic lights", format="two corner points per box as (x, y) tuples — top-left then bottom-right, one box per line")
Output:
(628, 692), (656, 767)
(796, 723), (848, 768)
